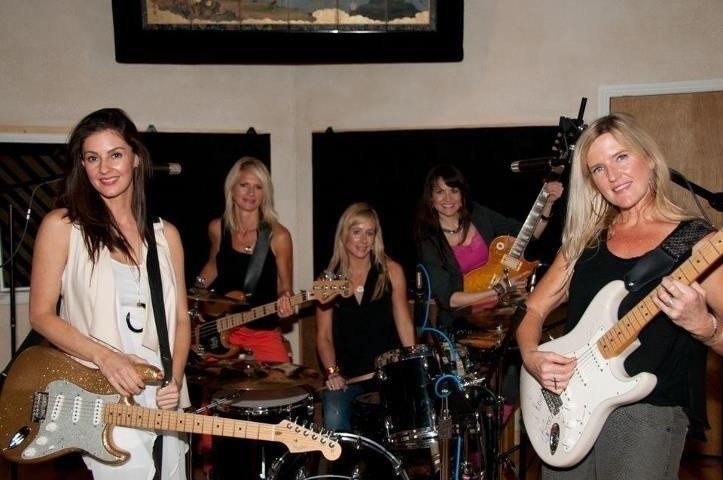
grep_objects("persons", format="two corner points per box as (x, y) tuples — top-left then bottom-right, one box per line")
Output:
(191, 157), (294, 320)
(316, 203), (415, 432)
(414, 165), (564, 316)
(515, 112), (723, 480)
(29, 106), (191, 480)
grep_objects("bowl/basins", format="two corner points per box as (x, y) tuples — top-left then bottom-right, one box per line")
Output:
(442, 222), (462, 234)
(232, 233), (255, 254)
(348, 263), (371, 294)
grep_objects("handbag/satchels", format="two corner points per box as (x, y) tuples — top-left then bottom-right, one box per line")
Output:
(696, 313), (717, 343)
(324, 365), (341, 377)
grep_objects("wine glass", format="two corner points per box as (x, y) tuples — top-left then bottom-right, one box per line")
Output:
(279, 309), (284, 315)
(551, 377), (560, 390)
(667, 297), (676, 308)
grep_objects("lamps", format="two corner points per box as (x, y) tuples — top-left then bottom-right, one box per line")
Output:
(297, 300), (327, 431)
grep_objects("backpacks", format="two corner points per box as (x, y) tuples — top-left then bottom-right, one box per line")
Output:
(267, 432), (411, 480)
(373, 342), (480, 449)
(212, 382), (315, 478)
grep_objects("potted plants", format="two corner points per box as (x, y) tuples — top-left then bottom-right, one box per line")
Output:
(111, 0), (464, 64)
